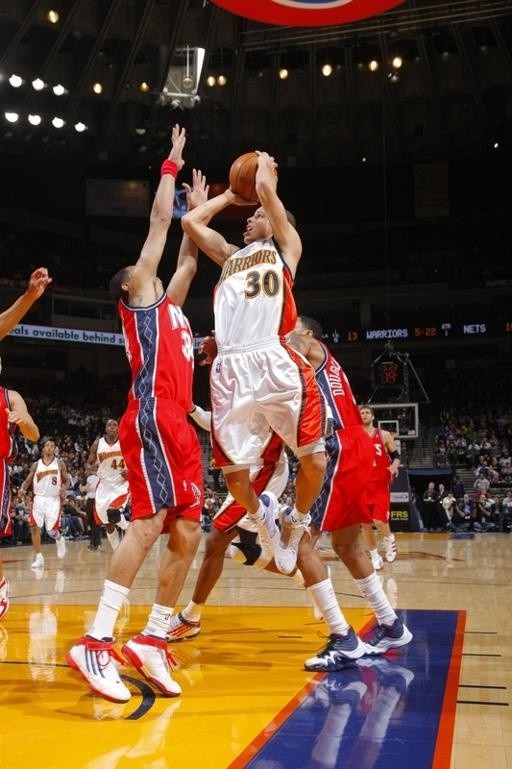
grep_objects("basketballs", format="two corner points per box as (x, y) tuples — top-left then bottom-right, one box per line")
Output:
(229, 152), (277, 203)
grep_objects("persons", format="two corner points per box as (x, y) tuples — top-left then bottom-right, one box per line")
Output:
(434, 407), (512, 452)
(8, 495), (31, 547)
(284, 316), (414, 673)
(68, 123), (210, 702)
(62, 444), (87, 486)
(88, 417), (130, 550)
(181, 149), (332, 575)
(8, 439), (40, 489)
(452, 476), (464, 498)
(19, 436), (69, 569)
(164, 400), (330, 642)
(202, 484), (223, 528)
(435, 444), (474, 469)
(62, 494), (89, 540)
(474, 453), (512, 533)
(0, 358), (39, 616)
(0, 267), (53, 346)
(434, 484), (448, 531)
(276, 483), (296, 512)
(458, 494), (474, 525)
(442, 491), (456, 527)
(79, 467), (103, 552)
(423, 483), (437, 533)
(360, 406), (400, 570)
(45, 402), (108, 442)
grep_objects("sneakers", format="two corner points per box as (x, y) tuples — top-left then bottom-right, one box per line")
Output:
(121, 632), (181, 691)
(306, 628), (366, 671)
(370, 547), (382, 570)
(154, 612), (200, 637)
(31, 558), (43, 580)
(1, 580), (9, 616)
(255, 507), (281, 552)
(66, 636), (128, 700)
(56, 536), (65, 558)
(276, 508), (311, 573)
(314, 565), (332, 621)
(361, 618), (416, 646)
(382, 535), (398, 563)
